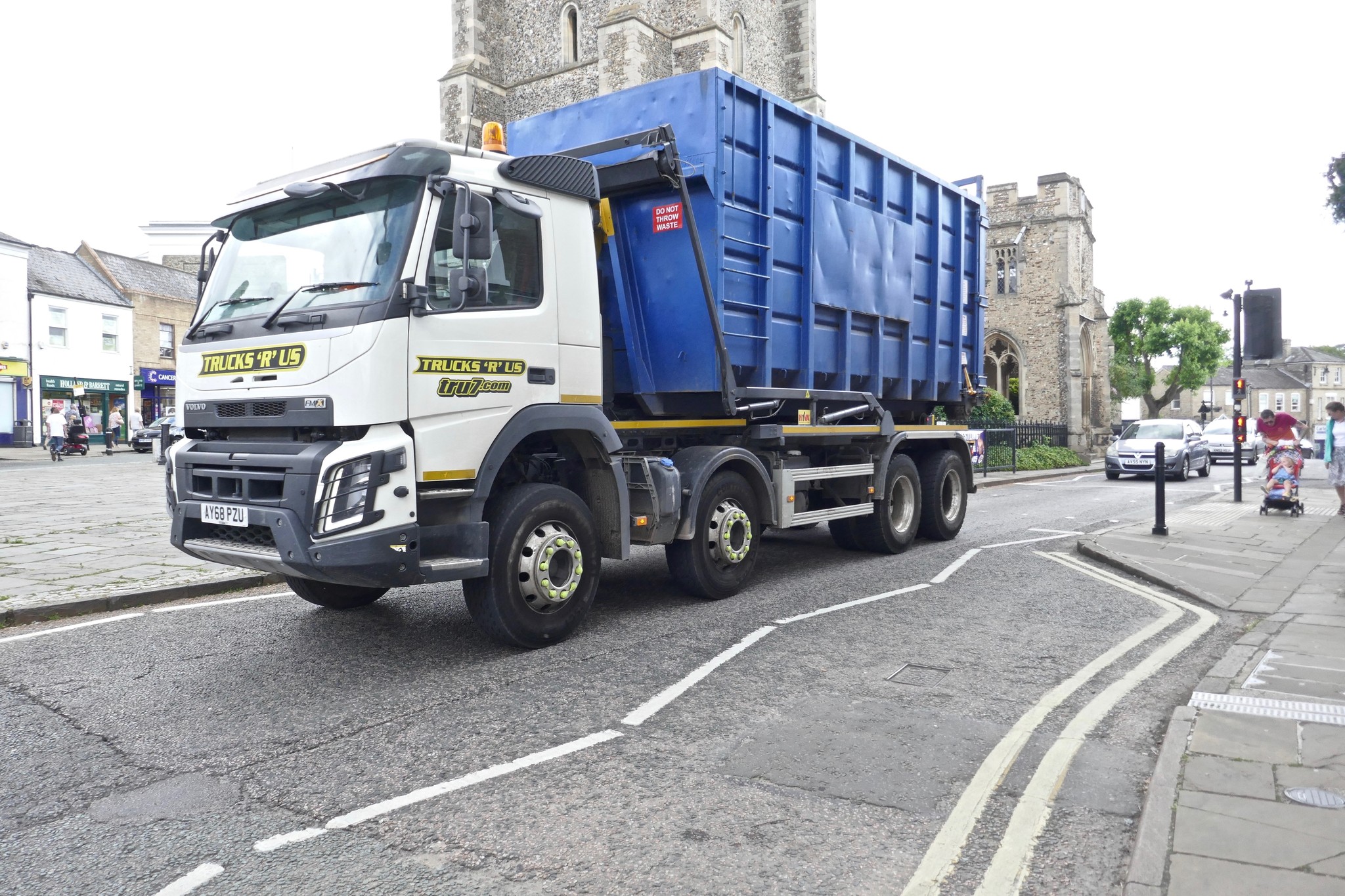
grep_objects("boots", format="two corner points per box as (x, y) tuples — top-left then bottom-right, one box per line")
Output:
(57, 453), (64, 462)
(51, 450), (59, 462)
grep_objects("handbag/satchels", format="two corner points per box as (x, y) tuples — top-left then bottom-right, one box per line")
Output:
(115, 412), (124, 424)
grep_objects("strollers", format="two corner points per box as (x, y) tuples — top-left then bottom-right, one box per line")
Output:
(1259, 445), (1304, 518)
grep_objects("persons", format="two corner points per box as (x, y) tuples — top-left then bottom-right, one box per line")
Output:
(1260, 458), (1295, 497)
(1325, 401), (1345, 516)
(128, 407), (145, 447)
(43, 407), (57, 450)
(108, 407), (122, 448)
(1256, 409), (1309, 509)
(46, 408), (69, 461)
(65, 404), (83, 426)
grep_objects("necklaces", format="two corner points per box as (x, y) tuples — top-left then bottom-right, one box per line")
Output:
(1335, 417), (1345, 424)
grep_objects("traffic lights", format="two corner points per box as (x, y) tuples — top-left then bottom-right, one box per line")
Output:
(1232, 416), (1247, 443)
(1231, 378), (1247, 399)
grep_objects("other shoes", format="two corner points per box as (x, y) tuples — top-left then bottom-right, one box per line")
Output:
(1282, 492), (1291, 497)
(1337, 504), (1345, 518)
(116, 445), (120, 448)
(111, 441), (114, 447)
(128, 440), (132, 447)
(1260, 485), (1269, 494)
(43, 445), (47, 450)
(50, 446), (54, 454)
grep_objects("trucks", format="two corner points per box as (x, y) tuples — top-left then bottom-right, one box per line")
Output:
(163, 67), (988, 652)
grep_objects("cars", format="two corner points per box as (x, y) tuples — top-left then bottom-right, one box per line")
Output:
(1105, 419), (1211, 482)
(132, 416), (185, 453)
(1201, 419), (1314, 465)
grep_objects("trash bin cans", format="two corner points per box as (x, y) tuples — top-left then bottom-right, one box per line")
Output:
(12, 420), (33, 448)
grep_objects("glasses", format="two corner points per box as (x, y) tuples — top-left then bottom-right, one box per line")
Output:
(1263, 421), (1273, 425)
(1328, 412), (1333, 416)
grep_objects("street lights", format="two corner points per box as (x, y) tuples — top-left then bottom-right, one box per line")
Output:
(1198, 373), (1212, 422)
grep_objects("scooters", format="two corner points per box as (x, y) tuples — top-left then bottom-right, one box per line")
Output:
(50, 419), (89, 456)
